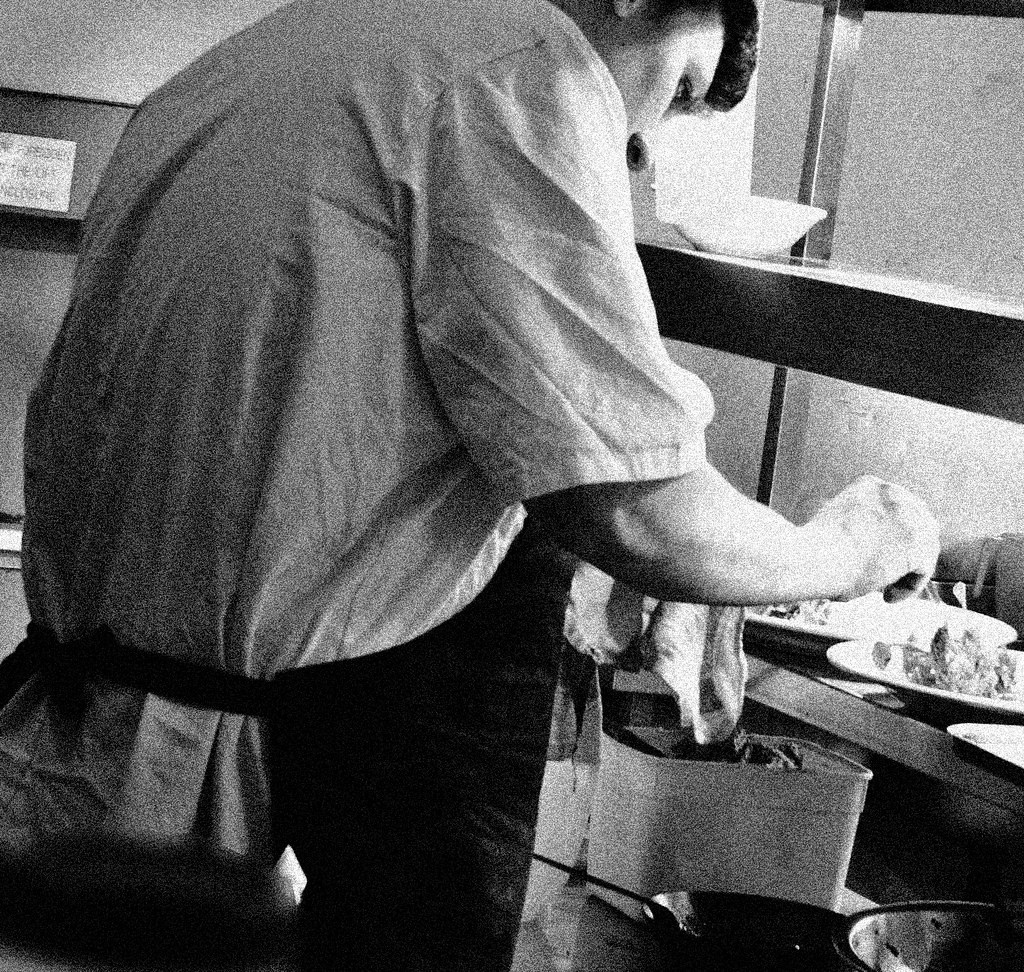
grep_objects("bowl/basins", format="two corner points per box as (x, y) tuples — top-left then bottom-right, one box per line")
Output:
(648, 176), (830, 261)
(642, 891), (846, 972)
(830, 902), (1024, 972)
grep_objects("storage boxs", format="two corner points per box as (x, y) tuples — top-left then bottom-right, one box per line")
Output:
(585, 725), (873, 920)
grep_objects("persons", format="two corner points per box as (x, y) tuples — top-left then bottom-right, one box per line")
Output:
(0, 0), (943, 972)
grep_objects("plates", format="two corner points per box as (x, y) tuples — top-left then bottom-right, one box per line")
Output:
(744, 590), (1018, 650)
(948, 723), (1024, 768)
(827, 640), (1024, 715)
